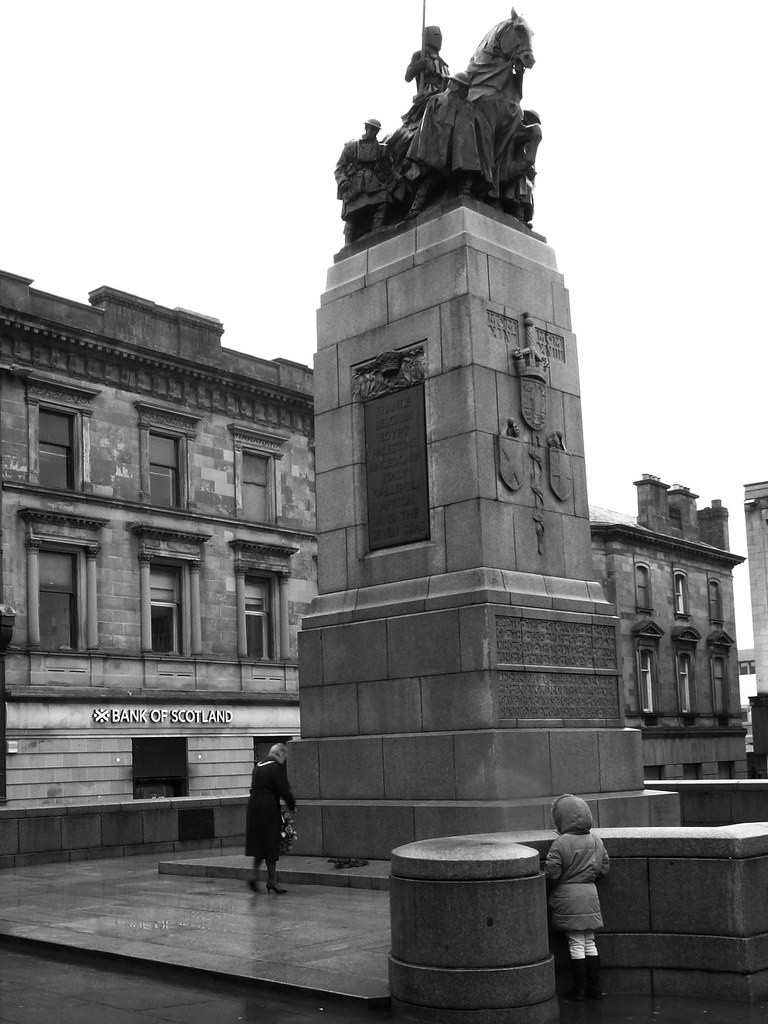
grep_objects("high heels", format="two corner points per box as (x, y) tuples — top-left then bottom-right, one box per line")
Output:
(265, 881), (288, 894)
(246, 877), (262, 893)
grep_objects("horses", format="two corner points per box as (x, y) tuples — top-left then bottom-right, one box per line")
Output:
(381, 7), (543, 199)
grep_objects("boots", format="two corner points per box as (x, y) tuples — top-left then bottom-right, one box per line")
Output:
(585, 955), (601, 1002)
(561, 955), (587, 1000)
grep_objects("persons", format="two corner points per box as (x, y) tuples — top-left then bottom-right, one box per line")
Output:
(516, 110), (542, 229)
(407, 72), (496, 221)
(405, 26), (449, 123)
(544, 793), (610, 1000)
(244, 743), (297, 895)
(334, 119), (387, 246)
